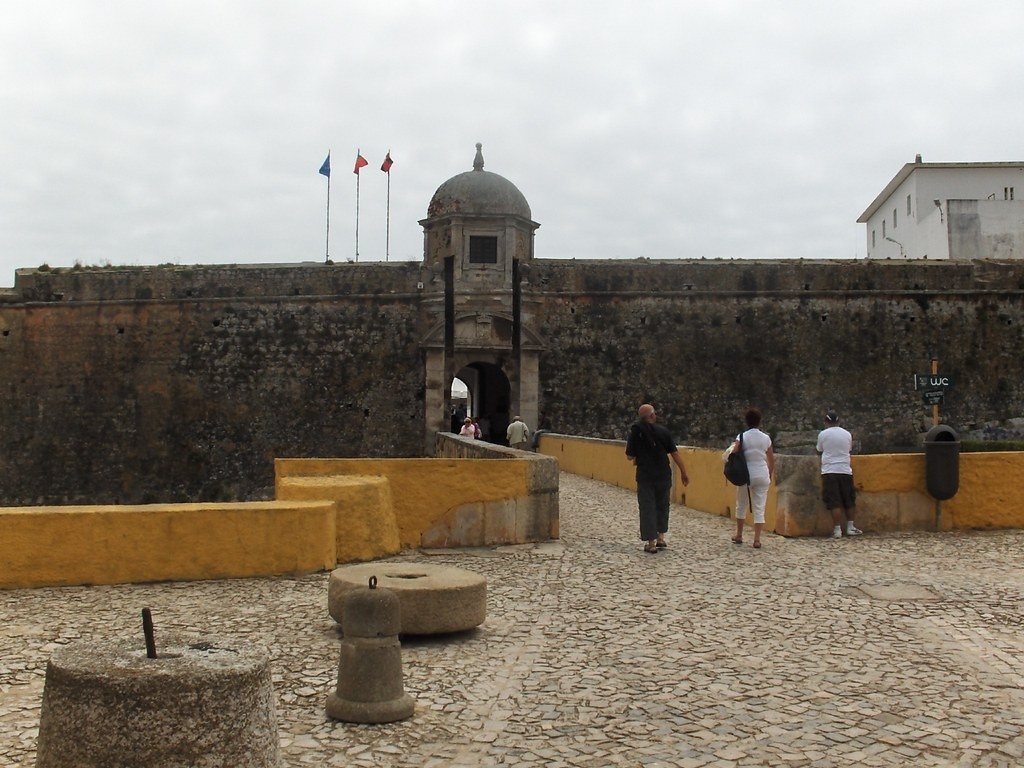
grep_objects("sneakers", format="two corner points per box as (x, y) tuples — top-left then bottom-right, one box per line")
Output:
(846, 527), (862, 535)
(833, 529), (842, 538)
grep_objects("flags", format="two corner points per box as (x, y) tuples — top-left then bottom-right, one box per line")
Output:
(353, 154), (368, 175)
(319, 154), (329, 178)
(380, 153), (393, 172)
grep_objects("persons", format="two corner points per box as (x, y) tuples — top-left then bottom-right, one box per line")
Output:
(470, 416), (490, 441)
(459, 417), (475, 440)
(816, 414), (862, 539)
(531, 410), (551, 448)
(625, 404), (689, 553)
(506, 415), (529, 450)
(728, 409), (774, 548)
(451, 414), (465, 433)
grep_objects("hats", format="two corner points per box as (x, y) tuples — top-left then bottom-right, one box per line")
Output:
(825, 410), (838, 423)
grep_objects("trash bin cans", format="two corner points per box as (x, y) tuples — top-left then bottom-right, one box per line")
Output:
(923, 425), (961, 500)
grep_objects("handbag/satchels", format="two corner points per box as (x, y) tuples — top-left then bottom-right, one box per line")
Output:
(724, 431), (750, 486)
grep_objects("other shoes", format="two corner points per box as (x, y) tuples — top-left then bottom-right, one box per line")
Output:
(656, 541), (667, 547)
(754, 540), (761, 548)
(732, 536), (742, 542)
(644, 543), (657, 553)
